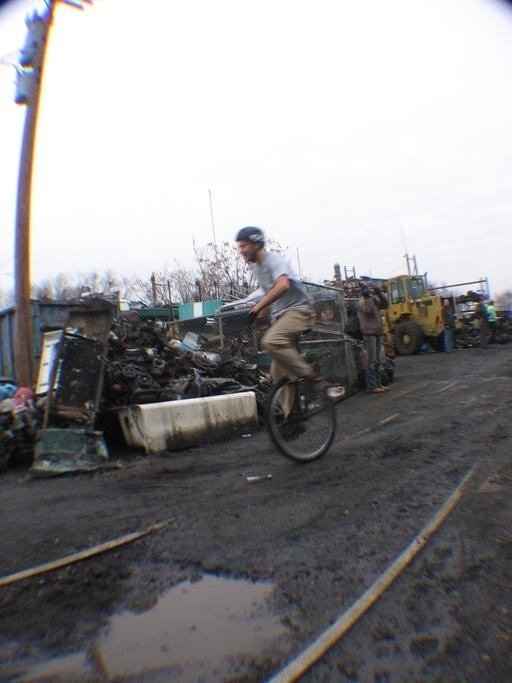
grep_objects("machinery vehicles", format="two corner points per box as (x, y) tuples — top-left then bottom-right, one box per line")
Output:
(455, 301), (507, 330)
(371, 274), (449, 356)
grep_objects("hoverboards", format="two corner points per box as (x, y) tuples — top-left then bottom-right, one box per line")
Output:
(264, 370), (337, 464)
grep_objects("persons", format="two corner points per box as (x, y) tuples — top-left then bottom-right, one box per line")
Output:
(356, 281), (391, 393)
(235, 226), (329, 439)
(441, 299), (455, 353)
(476, 299), (497, 349)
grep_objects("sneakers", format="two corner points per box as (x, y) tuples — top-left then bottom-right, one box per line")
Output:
(378, 385), (391, 390)
(368, 386), (386, 393)
(307, 384), (346, 410)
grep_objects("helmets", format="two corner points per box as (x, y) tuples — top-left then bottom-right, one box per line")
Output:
(235, 227), (264, 243)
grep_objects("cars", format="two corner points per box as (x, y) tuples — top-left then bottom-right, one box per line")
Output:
(215, 280), (340, 325)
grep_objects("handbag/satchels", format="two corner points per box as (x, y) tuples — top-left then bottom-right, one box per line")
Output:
(344, 316), (363, 339)
